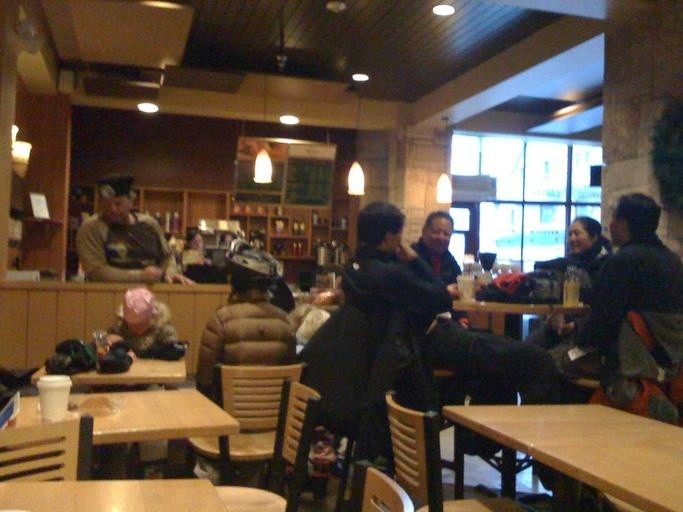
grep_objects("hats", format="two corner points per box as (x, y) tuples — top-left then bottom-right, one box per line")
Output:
(121, 286), (156, 325)
(94, 173), (135, 198)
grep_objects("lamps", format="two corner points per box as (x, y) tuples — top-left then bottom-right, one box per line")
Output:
(253, 11), (452, 207)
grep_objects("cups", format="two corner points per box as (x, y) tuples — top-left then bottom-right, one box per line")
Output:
(456, 274), (474, 303)
(93, 331), (108, 370)
(36, 374), (72, 423)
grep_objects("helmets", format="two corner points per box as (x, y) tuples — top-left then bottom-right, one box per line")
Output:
(224, 238), (284, 293)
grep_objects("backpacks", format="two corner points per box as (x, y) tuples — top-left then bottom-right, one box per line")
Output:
(474, 269), (565, 308)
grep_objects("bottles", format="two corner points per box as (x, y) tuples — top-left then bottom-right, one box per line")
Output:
(143, 202), (348, 256)
(564, 265), (580, 305)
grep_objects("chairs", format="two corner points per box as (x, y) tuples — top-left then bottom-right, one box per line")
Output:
(1, 283), (682, 512)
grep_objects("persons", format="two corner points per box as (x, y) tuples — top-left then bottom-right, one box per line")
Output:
(343, 200), (571, 403)
(411, 212), (470, 327)
(75, 175), (195, 286)
(95, 289), (178, 363)
(549, 192), (682, 373)
(218, 231), (238, 243)
(195, 238), (296, 405)
(527, 211), (610, 398)
(183, 229), (205, 254)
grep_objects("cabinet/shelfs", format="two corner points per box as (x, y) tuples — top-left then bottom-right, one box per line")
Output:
(69, 182), (347, 290)
